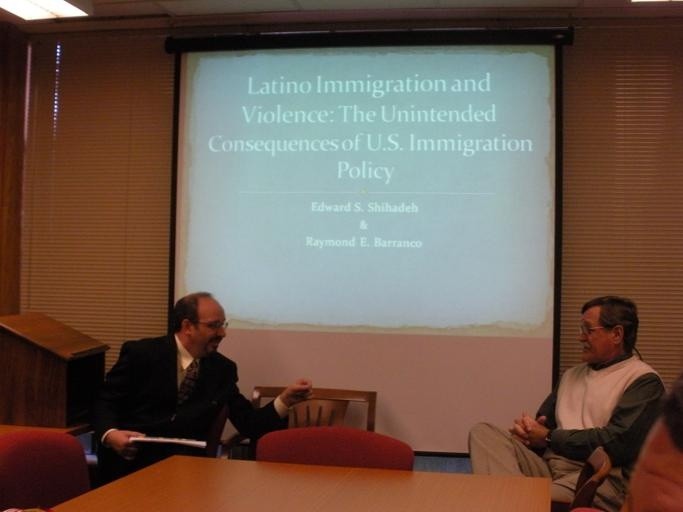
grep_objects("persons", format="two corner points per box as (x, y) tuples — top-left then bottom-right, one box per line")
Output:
(469, 296), (668, 509)
(618, 378), (683, 512)
(88, 291), (314, 489)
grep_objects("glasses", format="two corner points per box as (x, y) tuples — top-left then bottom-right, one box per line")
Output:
(199, 321), (228, 330)
(578, 323), (604, 335)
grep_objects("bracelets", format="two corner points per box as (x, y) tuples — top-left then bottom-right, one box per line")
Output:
(545, 431), (552, 448)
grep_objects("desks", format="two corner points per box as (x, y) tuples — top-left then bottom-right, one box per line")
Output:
(44, 452), (552, 512)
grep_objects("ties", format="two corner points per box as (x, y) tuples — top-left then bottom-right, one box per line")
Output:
(178, 360), (198, 404)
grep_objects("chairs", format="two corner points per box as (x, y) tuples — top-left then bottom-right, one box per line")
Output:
(0, 429), (90, 509)
(247, 383), (415, 470)
(569, 446), (612, 507)
